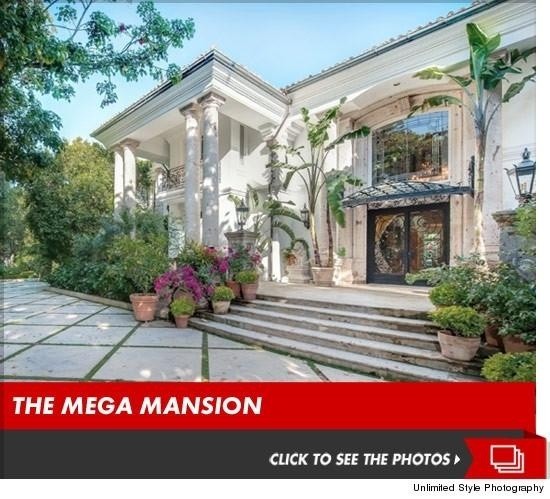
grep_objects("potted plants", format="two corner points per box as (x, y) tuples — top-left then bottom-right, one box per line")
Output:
(168, 286), (236, 329)
(123, 261), (157, 323)
(427, 260), (536, 365)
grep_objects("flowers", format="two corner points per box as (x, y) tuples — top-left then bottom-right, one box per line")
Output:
(154, 244), (264, 301)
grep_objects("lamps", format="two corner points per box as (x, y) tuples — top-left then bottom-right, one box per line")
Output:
(504, 147), (536, 211)
(300, 202), (310, 229)
(235, 198), (250, 230)
